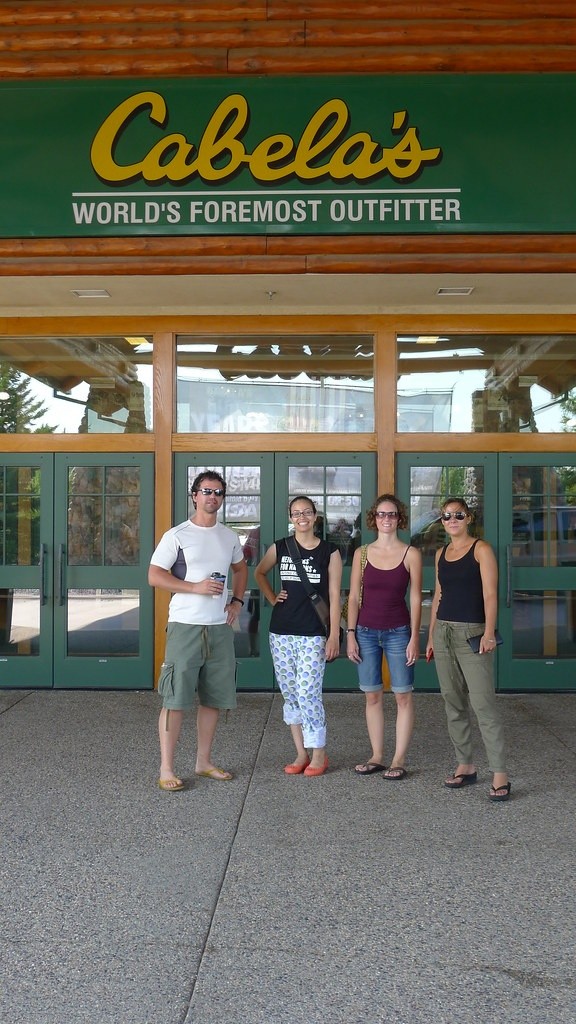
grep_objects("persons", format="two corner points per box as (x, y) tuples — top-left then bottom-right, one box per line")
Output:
(147, 471), (249, 791)
(425, 498), (512, 800)
(347, 494), (423, 780)
(253, 496), (342, 776)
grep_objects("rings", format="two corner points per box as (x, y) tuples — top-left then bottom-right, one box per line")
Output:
(488, 650), (492, 653)
(412, 658), (416, 661)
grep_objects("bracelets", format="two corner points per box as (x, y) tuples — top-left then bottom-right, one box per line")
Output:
(346, 629), (355, 633)
(230, 597), (244, 607)
(483, 636), (496, 643)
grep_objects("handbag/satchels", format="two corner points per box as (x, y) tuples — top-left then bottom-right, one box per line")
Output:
(342, 595), (362, 625)
(326, 627), (343, 663)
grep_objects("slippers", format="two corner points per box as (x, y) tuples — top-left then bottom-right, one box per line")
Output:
(159, 778), (185, 791)
(198, 767), (233, 781)
(383, 767), (408, 780)
(354, 761), (387, 775)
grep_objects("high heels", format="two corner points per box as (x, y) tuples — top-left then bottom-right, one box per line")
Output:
(489, 781), (510, 801)
(445, 772), (477, 789)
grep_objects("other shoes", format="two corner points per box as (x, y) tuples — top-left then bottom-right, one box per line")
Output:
(304, 757), (328, 776)
(285, 756), (310, 774)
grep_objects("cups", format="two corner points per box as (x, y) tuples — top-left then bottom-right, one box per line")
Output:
(211, 572), (226, 599)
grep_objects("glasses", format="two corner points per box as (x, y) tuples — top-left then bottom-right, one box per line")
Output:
(374, 512), (400, 520)
(195, 488), (225, 496)
(291, 510), (314, 518)
(441, 511), (469, 521)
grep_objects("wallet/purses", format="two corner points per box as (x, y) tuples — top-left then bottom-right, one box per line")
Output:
(466, 630), (503, 654)
